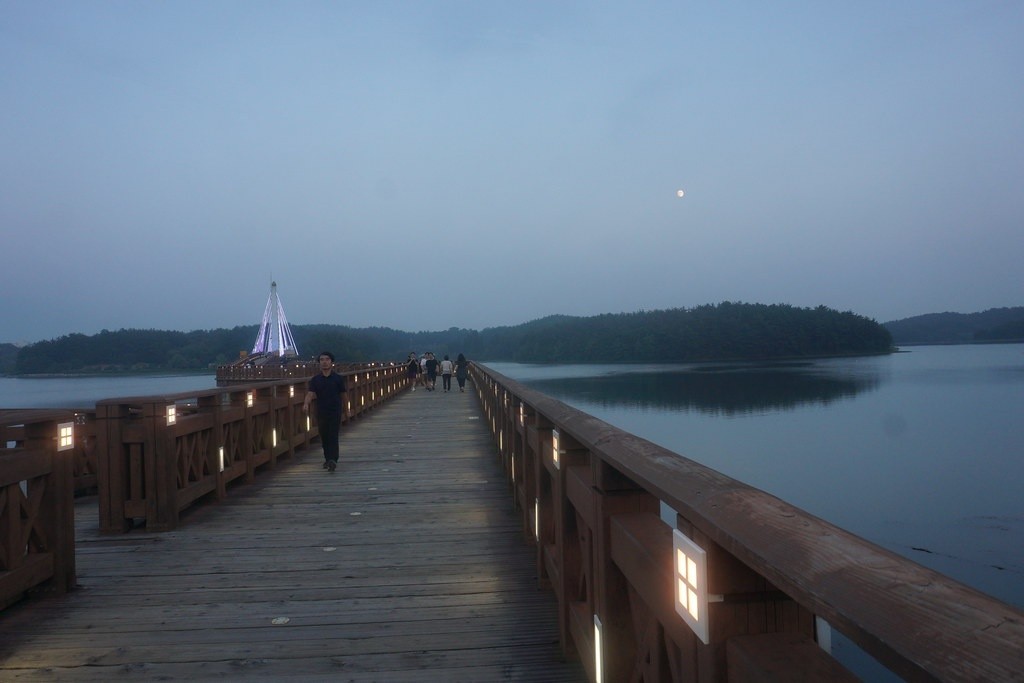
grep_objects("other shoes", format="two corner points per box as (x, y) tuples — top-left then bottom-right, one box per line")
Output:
(410, 387), (413, 391)
(460, 387), (464, 393)
(420, 383), (436, 392)
(412, 387), (416, 392)
(444, 389), (447, 393)
(447, 390), (450, 393)
(322, 460), (336, 472)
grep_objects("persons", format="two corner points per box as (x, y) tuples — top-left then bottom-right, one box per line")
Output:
(454, 353), (468, 392)
(440, 355), (455, 392)
(407, 351), (440, 392)
(302, 352), (350, 471)
